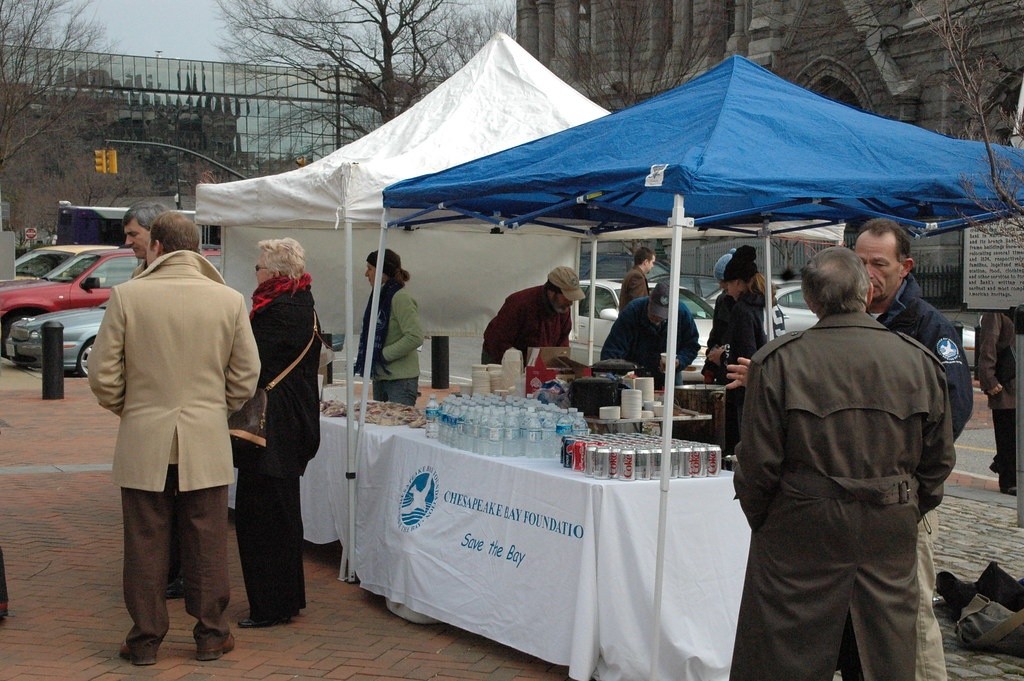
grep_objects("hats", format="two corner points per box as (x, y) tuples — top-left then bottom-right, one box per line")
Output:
(648, 281), (670, 318)
(723, 244), (758, 282)
(548, 267), (586, 300)
(712, 247), (736, 281)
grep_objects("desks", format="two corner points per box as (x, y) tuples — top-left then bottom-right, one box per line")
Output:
(227, 400), (751, 681)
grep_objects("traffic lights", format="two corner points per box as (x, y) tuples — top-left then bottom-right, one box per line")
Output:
(297, 156), (304, 169)
(107, 149), (118, 175)
(94, 149), (107, 175)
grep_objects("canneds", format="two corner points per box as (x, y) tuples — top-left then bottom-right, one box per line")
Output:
(561, 432), (722, 481)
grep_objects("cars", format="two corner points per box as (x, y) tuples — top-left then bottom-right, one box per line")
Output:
(705, 277), (978, 373)
(0, 245), (122, 288)
(571, 279), (725, 384)
(4, 298), (112, 377)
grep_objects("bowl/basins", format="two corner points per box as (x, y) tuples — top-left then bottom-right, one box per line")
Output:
(661, 353), (678, 364)
(459, 349), (521, 396)
(654, 405), (664, 416)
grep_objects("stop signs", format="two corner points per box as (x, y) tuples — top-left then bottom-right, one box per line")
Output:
(25, 228), (38, 240)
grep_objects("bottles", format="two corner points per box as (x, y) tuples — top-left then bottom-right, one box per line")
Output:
(425, 393), (589, 458)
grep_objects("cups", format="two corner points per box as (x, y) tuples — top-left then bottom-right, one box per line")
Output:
(620, 389), (643, 418)
(599, 406), (620, 420)
(635, 376), (655, 411)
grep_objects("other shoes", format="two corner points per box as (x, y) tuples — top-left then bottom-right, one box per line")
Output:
(989, 461), (999, 474)
(999, 484), (1017, 495)
(163, 578), (186, 599)
(0, 602), (9, 617)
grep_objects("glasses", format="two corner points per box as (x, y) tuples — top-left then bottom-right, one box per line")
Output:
(255, 265), (269, 271)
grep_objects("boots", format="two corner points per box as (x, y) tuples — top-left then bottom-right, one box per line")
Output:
(937, 559), (1024, 621)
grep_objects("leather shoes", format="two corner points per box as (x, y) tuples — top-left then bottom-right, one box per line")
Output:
(197, 632), (235, 661)
(119, 640), (156, 664)
(238, 607), (303, 627)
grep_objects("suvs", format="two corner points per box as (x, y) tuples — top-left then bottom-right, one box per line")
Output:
(0, 246), (223, 363)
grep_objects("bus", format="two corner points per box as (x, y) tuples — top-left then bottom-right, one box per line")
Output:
(55, 200), (221, 248)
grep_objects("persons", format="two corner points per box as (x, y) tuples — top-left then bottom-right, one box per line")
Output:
(619, 247), (656, 312)
(729, 246), (957, 681)
(481, 266), (586, 365)
(600, 283), (702, 389)
(122, 201), (184, 599)
(978, 312), (1017, 496)
(232, 237), (321, 628)
(355, 249), (425, 407)
(702, 245), (776, 472)
(725, 218), (973, 681)
(87, 211), (262, 664)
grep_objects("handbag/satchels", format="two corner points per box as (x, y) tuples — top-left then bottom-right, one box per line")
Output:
(227, 386), (270, 450)
(955, 594), (1024, 657)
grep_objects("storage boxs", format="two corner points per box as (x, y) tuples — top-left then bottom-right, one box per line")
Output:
(525, 345), (591, 394)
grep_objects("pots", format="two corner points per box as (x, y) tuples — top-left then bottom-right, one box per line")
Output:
(674, 384), (726, 452)
(591, 358), (638, 375)
(570, 377), (616, 417)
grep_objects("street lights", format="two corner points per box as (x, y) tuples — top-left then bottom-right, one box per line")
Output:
(318, 62), (342, 150)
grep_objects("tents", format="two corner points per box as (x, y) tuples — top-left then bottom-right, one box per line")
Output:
(194, 33), (614, 581)
(339, 56), (1024, 681)
